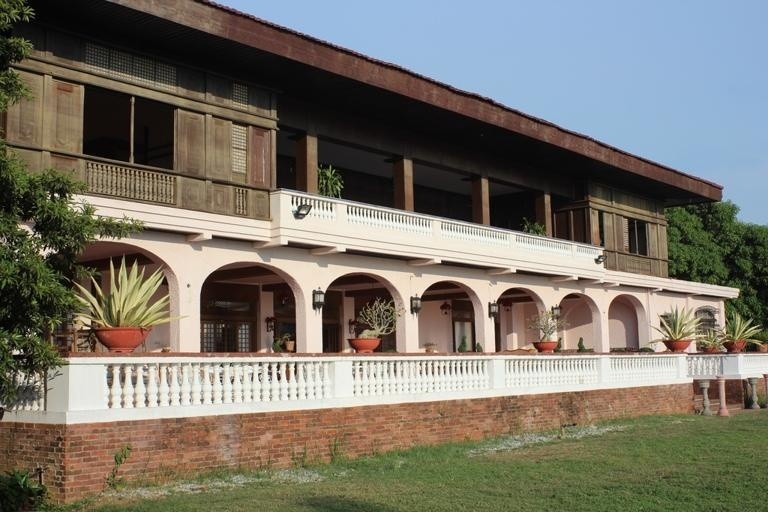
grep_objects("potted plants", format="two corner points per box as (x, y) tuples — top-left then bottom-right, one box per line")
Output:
(423, 342), (437, 354)
(271, 332), (296, 353)
(63, 253), (175, 352)
(526, 306), (566, 354)
(343, 296), (406, 354)
(648, 303), (768, 355)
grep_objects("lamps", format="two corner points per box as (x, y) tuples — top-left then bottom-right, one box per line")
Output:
(594, 254), (607, 264)
(291, 202), (313, 221)
(410, 294), (424, 318)
(550, 304), (562, 321)
(501, 298), (513, 312)
(440, 301), (453, 318)
(486, 300), (501, 320)
(310, 286), (327, 314)
